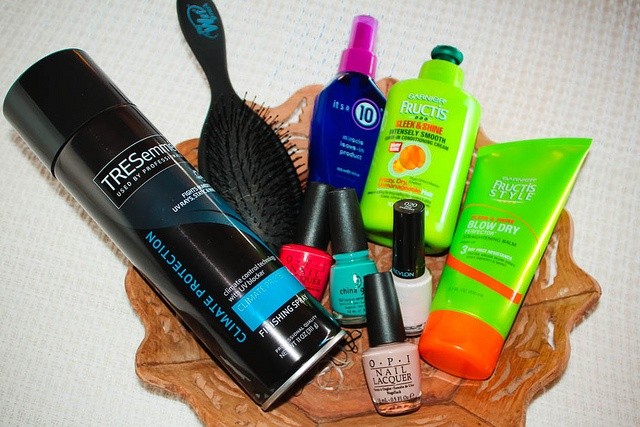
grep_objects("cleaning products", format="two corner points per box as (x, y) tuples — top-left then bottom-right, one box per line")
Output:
(309, 15), (386, 202)
(359, 44), (481, 256)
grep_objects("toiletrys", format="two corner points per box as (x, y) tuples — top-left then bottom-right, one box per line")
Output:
(389, 200), (432, 336)
(280, 184), (331, 303)
(325, 189), (378, 325)
(419, 137), (594, 381)
(360, 273), (422, 416)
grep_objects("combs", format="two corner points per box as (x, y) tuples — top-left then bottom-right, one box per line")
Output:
(175, 0), (310, 256)
